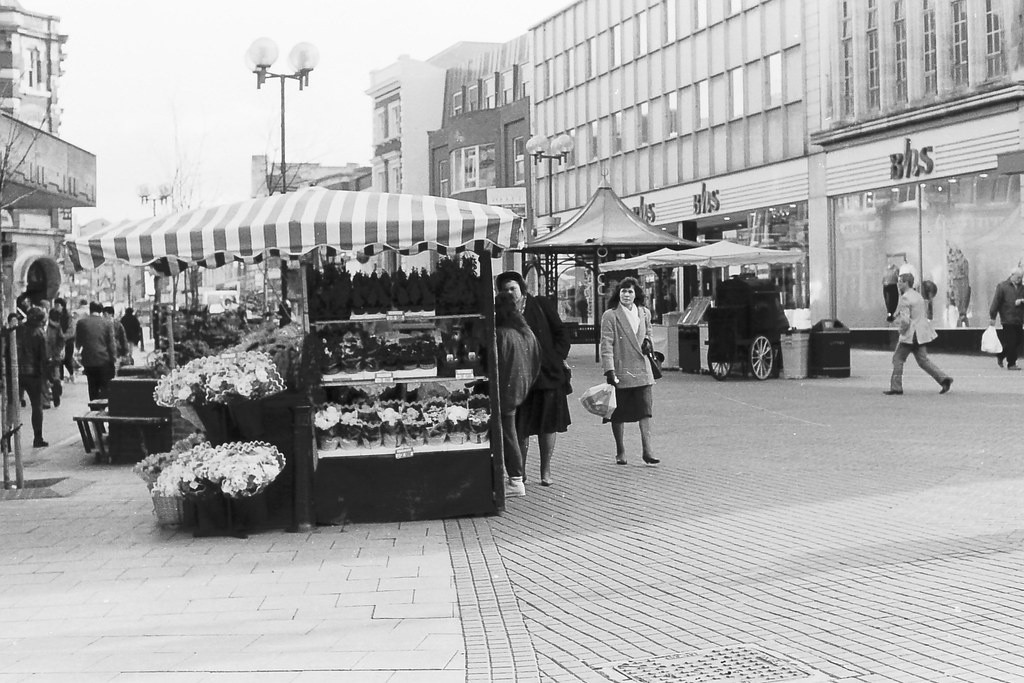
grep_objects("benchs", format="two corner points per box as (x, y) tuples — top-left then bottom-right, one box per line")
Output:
(74, 398), (169, 464)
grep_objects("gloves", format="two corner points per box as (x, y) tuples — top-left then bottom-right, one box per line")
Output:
(605, 370), (619, 385)
(641, 339), (652, 354)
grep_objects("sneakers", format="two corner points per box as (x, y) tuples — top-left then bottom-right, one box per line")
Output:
(493, 476), (526, 498)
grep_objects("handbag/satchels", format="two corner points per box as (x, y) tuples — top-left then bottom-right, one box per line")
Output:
(561, 359), (572, 382)
(647, 351), (665, 379)
(981, 326), (1003, 354)
(579, 376), (619, 420)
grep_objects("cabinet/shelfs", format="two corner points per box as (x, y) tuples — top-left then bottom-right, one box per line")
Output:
(299, 248), (507, 527)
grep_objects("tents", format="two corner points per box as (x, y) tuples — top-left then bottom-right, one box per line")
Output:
(65, 185), (522, 339)
(597, 239), (803, 278)
(525, 179), (712, 363)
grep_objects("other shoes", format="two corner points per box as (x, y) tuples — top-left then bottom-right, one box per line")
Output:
(940, 377), (953, 394)
(21, 399), (26, 407)
(1007, 365), (1021, 370)
(52, 387), (61, 407)
(42, 403), (51, 409)
(996, 357), (1004, 368)
(33, 439), (48, 448)
(643, 455), (660, 464)
(884, 390), (904, 394)
(615, 454), (627, 464)
(542, 476), (553, 485)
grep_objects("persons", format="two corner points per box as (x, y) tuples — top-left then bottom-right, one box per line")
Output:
(8, 297), (141, 409)
(493, 292), (542, 498)
(989, 268), (1024, 370)
(76, 301), (117, 433)
(600, 277), (660, 464)
(496, 271), (571, 486)
(882, 252), (920, 320)
(6, 307), (48, 448)
(883, 273), (953, 395)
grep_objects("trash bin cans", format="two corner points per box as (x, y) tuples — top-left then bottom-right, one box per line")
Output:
(809, 318), (851, 377)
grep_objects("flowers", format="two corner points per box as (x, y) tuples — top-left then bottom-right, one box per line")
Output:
(134, 351), (286, 497)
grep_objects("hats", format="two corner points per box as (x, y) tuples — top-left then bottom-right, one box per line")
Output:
(496, 270), (524, 293)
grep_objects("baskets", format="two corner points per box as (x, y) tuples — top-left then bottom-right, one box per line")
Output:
(152, 490), (196, 524)
